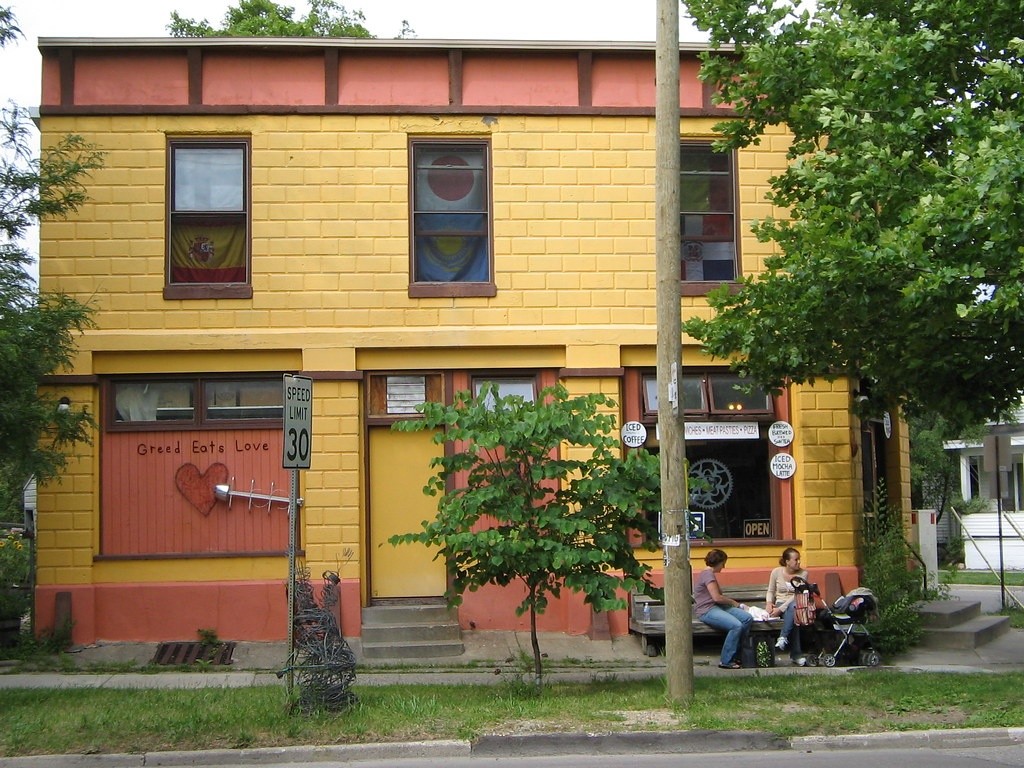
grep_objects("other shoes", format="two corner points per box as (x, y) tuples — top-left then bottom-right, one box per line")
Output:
(719, 661), (740, 669)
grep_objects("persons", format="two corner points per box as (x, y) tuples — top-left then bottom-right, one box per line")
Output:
(766, 548), (809, 667)
(695, 549), (752, 669)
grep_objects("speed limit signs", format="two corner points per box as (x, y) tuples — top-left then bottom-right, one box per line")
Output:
(282, 374), (314, 471)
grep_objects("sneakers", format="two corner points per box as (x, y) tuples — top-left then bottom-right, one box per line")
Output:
(793, 657), (808, 667)
(774, 636), (789, 650)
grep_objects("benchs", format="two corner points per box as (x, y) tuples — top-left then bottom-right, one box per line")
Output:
(629, 583), (821, 655)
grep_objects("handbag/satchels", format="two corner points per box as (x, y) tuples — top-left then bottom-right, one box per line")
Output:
(793, 585), (817, 626)
(755, 639), (775, 667)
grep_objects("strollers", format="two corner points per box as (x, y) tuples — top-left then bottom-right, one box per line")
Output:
(790, 575), (885, 667)
